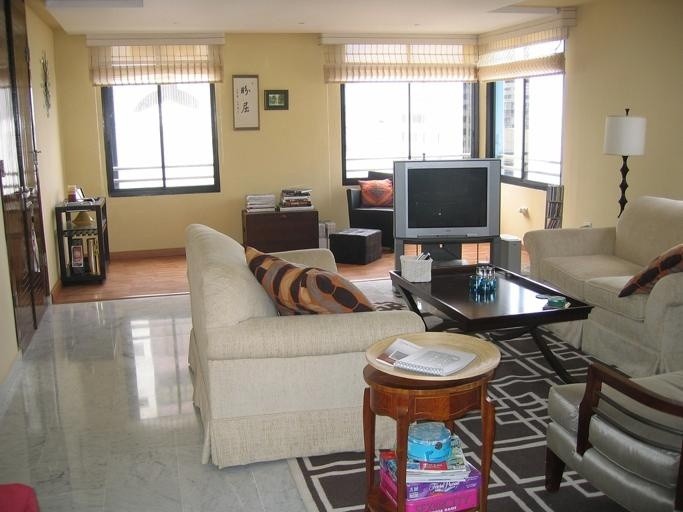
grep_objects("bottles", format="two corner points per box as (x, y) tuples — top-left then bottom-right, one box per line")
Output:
(86, 238), (97, 275)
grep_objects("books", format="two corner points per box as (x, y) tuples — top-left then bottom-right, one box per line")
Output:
(245, 187), (315, 213)
(94, 237), (101, 276)
(374, 434), (470, 482)
(86, 238), (95, 276)
(375, 339), (421, 368)
(393, 344), (476, 376)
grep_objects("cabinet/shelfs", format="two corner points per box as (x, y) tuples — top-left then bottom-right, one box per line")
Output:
(238, 209), (320, 254)
(54, 197), (109, 289)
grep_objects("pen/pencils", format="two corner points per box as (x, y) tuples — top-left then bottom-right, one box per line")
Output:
(416, 252), (430, 261)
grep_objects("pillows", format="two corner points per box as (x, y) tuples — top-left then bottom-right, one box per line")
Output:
(615, 242), (681, 300)
(357, 178), (392, 207)
(244, 243), (375, 317)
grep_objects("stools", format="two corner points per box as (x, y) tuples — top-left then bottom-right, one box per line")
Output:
(328, 228), (382, 266)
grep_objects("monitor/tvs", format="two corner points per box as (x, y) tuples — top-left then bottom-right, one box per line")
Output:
(393, 158), (501, 242)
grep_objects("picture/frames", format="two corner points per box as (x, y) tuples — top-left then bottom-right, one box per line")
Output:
(231, 74), (261, 131)
(264, 89), (288, 110)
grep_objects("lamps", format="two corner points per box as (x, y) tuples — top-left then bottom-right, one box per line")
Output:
(599, 107), (650, 220)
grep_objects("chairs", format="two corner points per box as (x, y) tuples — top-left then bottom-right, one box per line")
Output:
(346, 171), (394, 254)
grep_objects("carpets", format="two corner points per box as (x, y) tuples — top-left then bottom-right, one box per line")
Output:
(289, 302), (631, 512)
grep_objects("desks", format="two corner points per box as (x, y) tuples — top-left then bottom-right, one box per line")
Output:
(359, 362), (498, 510)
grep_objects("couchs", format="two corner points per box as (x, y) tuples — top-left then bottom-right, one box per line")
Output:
(522, 198), (682, 378)
(542, 362), (681, 511)
(181, 222), (428, 472)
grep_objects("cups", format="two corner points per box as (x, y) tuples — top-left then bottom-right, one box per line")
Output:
(66, 185), (76, 202)
(468, 264), (496, 293)
(468, 293), (496, 304)
